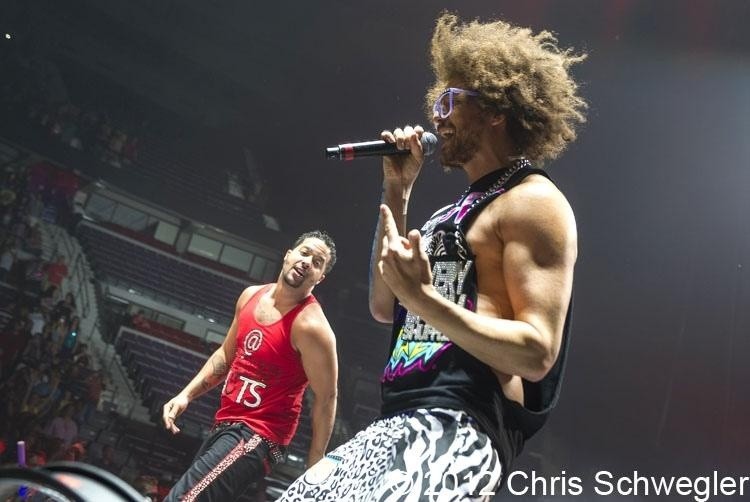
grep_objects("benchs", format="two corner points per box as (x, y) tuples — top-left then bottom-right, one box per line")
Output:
(0, 54), (351, 502)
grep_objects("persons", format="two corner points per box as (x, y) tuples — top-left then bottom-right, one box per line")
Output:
(273, 9), (589, 502)
(162, 228), (338, 502)
(0, 77), (159, 502)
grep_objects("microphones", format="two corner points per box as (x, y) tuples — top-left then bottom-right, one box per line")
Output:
(324, 131), (441, 158)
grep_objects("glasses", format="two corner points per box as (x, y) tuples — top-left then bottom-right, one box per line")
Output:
(432, 87), (480, 129)
